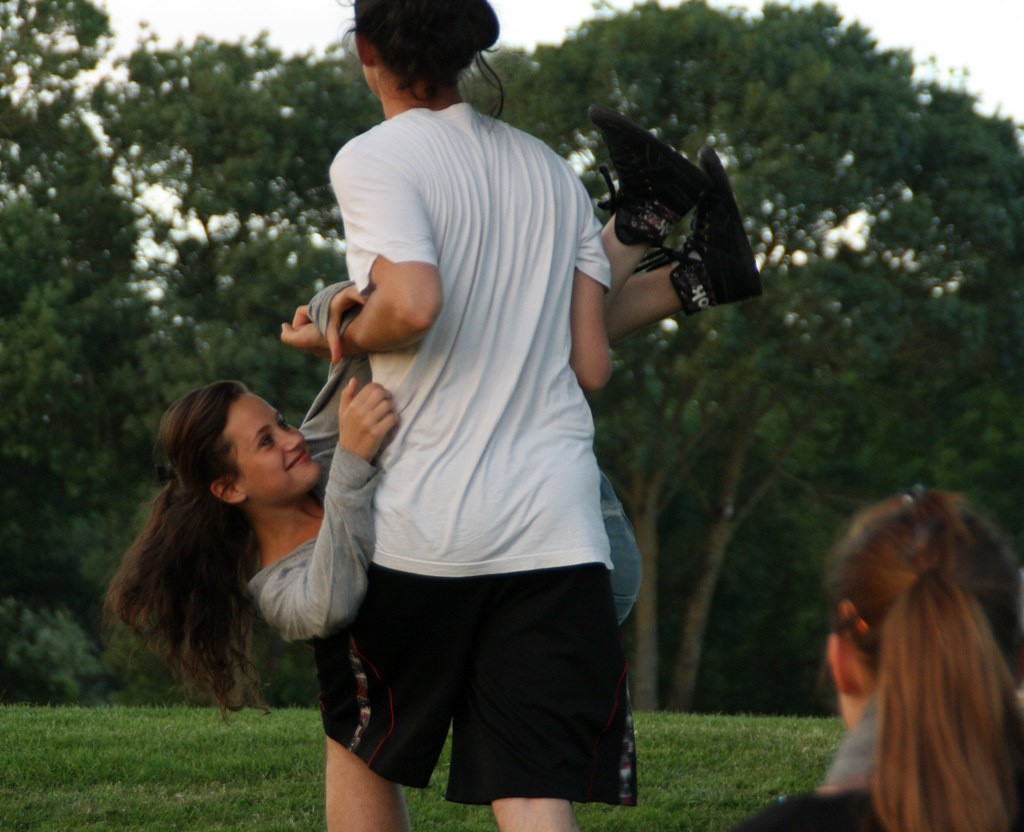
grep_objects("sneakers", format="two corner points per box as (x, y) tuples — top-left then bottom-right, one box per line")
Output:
(586, 105), (705, 249)
(636, 146), (763, 318)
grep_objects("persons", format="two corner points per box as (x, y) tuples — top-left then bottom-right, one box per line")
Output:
(102, 101), (759, 717)
(736, 486), (1024, 832)
(278, 1), (638, 831)
